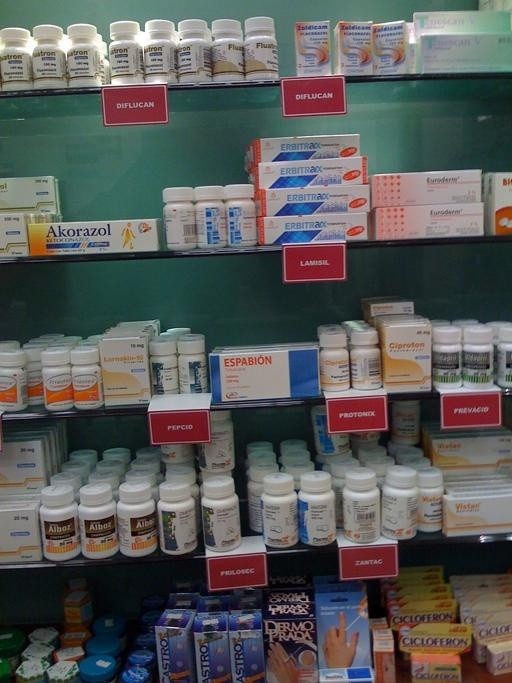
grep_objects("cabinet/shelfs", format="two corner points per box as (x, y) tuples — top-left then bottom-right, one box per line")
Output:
(0, 0), (512, 683)
